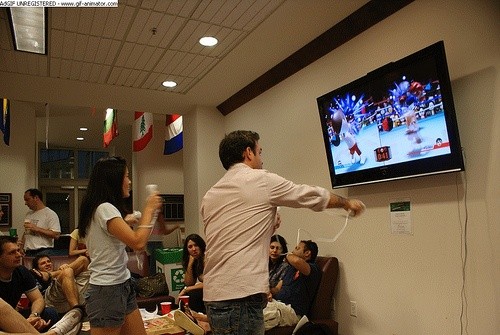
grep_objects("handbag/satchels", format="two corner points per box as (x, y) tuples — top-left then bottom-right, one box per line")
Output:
(137, 272), (168, 298)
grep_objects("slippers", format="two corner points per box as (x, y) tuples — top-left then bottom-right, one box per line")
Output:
(30, 268), (51, 289)
(178, 300), (192, 315)
(174, 310), (205, 335)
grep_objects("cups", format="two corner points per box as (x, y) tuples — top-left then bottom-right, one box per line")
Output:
(23, 219), (32, 230)
(160, 302), (172, 316)
(20, 294), (30, 308)
(9, 228), (17, 238)
(179, 295), (189, 312)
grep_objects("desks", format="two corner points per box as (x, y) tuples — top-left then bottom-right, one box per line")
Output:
(82, 307), (194, 333)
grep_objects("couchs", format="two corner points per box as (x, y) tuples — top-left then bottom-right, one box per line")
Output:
(279, 256), (340, 335)
(23, 251), (175, 319)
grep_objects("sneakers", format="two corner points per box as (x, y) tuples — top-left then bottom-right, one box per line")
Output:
(49, 308), (84, 335)
(62, 321), (81, 335)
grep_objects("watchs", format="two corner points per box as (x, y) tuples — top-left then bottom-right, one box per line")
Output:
(34, 313), (40, 317)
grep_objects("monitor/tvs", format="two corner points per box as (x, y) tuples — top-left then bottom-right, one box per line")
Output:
(316, 40), (466, 189)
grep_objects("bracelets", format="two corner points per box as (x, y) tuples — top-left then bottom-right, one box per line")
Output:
(184, 286), (187, 292)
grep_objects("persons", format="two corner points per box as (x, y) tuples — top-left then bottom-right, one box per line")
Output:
(0, 298), (83, 335)
(202, 129), (365, 335)
(146, 210), (181, 259)
(174, 240), (322, 335)
(69, 226), (91, 262)
(78, 155), (164, 335)
(177, 233), (205, 313)
(31, 254), (89, 308)
(0, 235), (55, 333)
(20, 188), (61, 257)
(268, 235), (290, 302)
